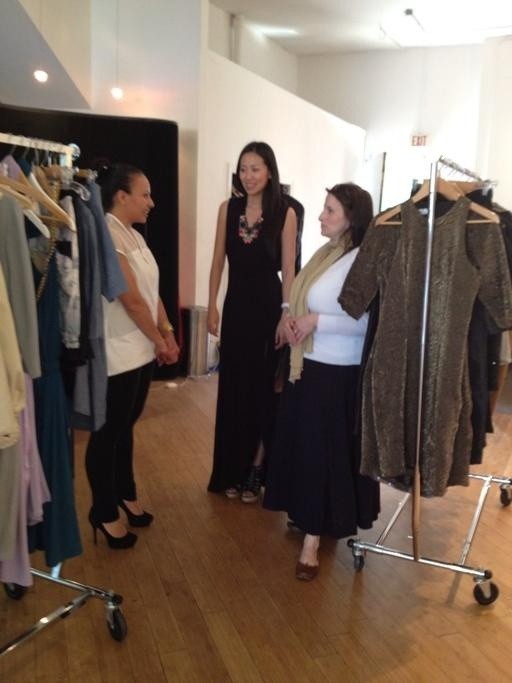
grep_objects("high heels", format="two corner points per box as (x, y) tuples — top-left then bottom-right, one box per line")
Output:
(89, 509), (138, 550)
(225, 477), (245, 499)
(241, 464), (263, 504)
(118, 496), (154, 527)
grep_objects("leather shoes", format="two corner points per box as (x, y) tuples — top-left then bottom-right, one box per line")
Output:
(286, 519), (304, 532)
(296, 551), (320, 581)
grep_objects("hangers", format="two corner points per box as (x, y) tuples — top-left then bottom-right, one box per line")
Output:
(375, 155), (500, 227)
(0, 132), (97, 239)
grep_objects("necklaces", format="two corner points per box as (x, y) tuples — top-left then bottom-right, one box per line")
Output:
(237, 194), (265, 244)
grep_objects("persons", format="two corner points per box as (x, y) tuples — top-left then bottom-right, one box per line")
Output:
(84, 163), (181, 549)
(265, 182), (381, 581)
(206, 141), (297, 504)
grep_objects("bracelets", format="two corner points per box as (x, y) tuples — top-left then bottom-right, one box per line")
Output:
(158, 322), (175, 333)
(280, 302), (289, 309)
